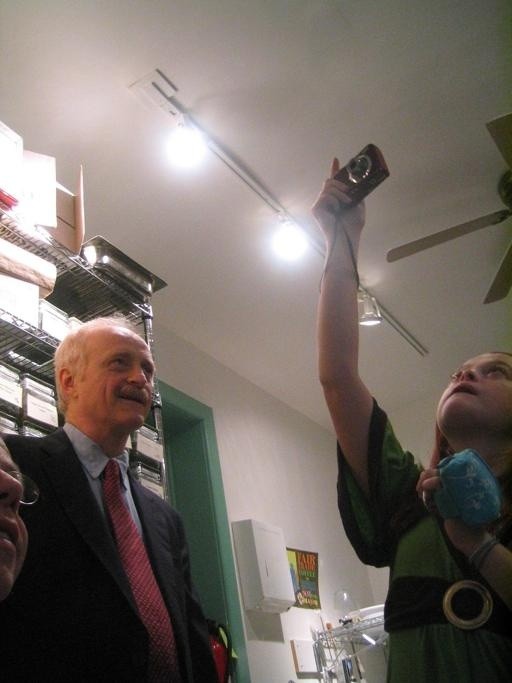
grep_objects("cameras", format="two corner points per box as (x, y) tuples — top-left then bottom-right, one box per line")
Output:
(332, 144), (390, 208)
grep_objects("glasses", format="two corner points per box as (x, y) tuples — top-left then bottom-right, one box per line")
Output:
(2, 466), (42, 507)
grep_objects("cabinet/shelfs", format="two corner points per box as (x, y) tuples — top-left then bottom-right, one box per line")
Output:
(0, 202), (166, 504)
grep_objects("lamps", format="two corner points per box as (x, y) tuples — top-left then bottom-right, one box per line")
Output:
(358, 290), (383, 327)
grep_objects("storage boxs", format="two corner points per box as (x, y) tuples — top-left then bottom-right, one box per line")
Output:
(13, 150), (84, 254)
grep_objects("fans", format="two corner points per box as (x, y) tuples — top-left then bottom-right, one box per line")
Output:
(386, 114), (512, 304)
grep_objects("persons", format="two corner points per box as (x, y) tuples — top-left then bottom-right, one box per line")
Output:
(2, 314), (233, 683)
(1, 436), (30, 603)
(312, 156), (511, 683)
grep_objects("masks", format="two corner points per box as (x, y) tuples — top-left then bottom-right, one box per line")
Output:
(436, 446), (507, 529)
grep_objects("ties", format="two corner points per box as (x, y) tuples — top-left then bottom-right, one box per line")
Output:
(100, 458), (182, 682)
